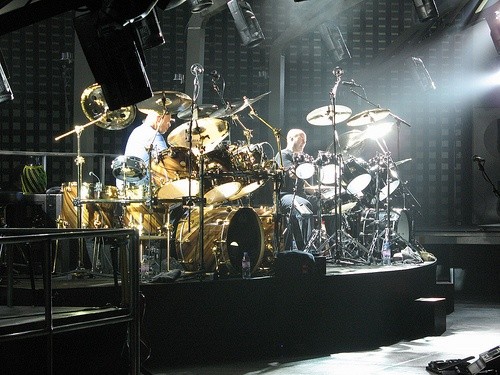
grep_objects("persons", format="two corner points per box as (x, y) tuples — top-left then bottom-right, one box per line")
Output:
(274, 128), (329, 256)
(116, 113), (173, 232)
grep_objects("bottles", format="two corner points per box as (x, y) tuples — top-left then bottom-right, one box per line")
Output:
(382, 240), (392, 266)
(242, 252), (252, 279)
(141, 255), (149, 281)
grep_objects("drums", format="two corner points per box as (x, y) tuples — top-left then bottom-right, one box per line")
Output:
(341, 154), (371, 196)
(232, 145), (273, 201)
(110, 155), (146, 184)
(363, 161), (401, 203)
(60, 181), (119, 229)
(322, 192), (358, 213)
(198, 149), (241, 202)
(147, 147), (203, 204)
(119, 179), (166, 236)
(315, 149), (341, 185)
(167, 117), (232, 158)
(174, 204), (265, 273)
(257, 206), (284, 263)
(290, 151), (315, 179)
(360, 207), (412, 256)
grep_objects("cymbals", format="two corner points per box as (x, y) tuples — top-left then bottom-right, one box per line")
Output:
(228, 88), (273, 118)
(138, 92), (193, 114)
(307, 105), (354, 125)
(80, 83), (138, 131)
(177, 103), (219, 119)
(346, 107), (391, 127)
(370, 157), (413, 174)
(210, 102), (240, 121)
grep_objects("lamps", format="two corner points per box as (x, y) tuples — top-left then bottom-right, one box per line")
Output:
(72, 0), (214, 110)
(318, 22), (352, 65)
(413, 0), (439, 23)
(227, 0), (265, 48)
(408, 58), (436, 94)
